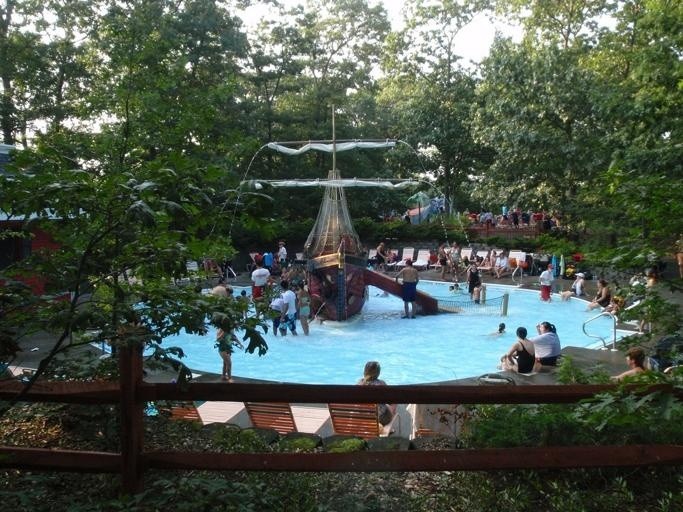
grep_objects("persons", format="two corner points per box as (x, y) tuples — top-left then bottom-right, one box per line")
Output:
(217, 328), (244, 383)
(501, 328), (535, 373)
(357, 362), (398, 426)
(528, 322), (561, 365)
(611, 347), (648, 384)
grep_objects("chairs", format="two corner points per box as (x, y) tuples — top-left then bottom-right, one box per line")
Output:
(154, 400), (246, 427)
(243, 401), (333, 435)
(163, 252), (309, 278)
(366, 245), (536, 282)
(327, 402), (419, 439)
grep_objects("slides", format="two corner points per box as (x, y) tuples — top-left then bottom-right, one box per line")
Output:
(363, 270), (438, 314)
